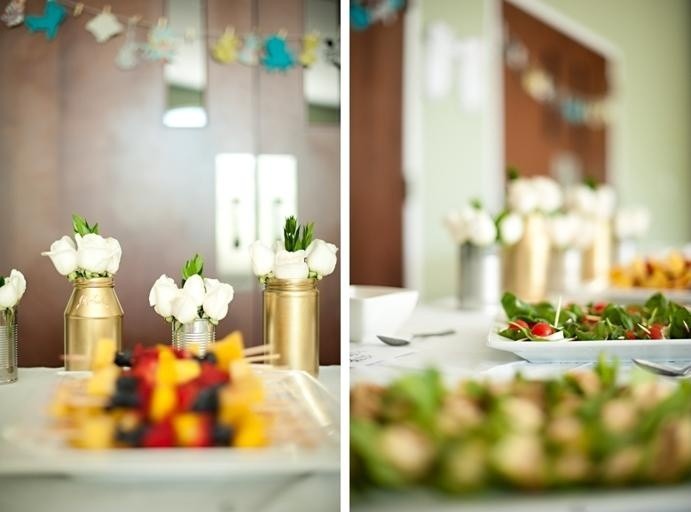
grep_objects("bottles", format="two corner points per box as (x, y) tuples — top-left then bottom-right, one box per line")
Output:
(0, 308), (19, 383)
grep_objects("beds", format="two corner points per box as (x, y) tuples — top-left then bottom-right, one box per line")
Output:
(0, 365), (342, 511)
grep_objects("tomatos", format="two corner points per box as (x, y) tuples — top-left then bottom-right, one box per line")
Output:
(531, 322), (555, 335)
(510, 318), (527, 328)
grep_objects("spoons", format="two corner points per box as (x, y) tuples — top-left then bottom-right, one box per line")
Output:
(377, 329), (456, 344)
(631, 357), (691, 379)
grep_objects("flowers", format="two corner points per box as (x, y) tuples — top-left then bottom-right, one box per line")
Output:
(252, 216), (338, 281)
(0, 269), (27, 324)
(43, 214), (121, 277)
(149, 255), (234, 332)
(446, 167), (650, 242)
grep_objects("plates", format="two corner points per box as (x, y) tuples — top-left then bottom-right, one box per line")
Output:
(349, 283), (419, 343)
(487, 307), (690, 362)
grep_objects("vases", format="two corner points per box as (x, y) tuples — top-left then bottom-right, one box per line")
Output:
(459, 217), (630, 301)
(0, 320), (19, 382)
